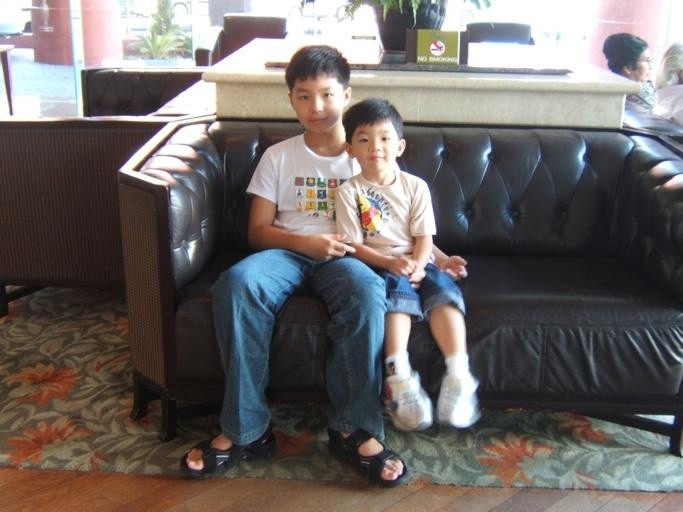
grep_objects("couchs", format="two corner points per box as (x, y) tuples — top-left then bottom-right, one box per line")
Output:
(118, 115), (683, 457)
(624, 102), (683, 141)
(1, 118), (168, 316)
(78, 67), (202, 116)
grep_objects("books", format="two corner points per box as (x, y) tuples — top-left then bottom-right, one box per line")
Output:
(415, 28), (461, 68)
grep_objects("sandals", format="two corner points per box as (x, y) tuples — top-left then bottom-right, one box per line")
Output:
(180, 429), (274, 478)
(328, 429), (409, 487)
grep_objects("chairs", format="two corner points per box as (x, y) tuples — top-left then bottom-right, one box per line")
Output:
(461, 22), (535, 63)
(209, 15), (288, 66)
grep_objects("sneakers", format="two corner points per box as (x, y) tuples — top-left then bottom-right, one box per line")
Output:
(382, 371), (433, 431)
(435, 373), (479, 429)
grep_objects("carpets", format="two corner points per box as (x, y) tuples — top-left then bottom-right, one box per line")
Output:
(0, 281), (683, 493)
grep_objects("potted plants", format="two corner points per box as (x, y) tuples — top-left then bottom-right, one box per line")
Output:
(301, 0), (493, 53)
(131, 24), (193, 66)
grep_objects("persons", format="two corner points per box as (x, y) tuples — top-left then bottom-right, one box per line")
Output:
(334, 98), (484, 435)
(179, 43), (469, 491)
(600, 31), (656, 111)
(647, 43), (681, 129)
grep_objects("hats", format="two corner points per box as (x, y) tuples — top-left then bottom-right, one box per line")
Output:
(664, 43), (683, 71)
(603, 33), (648, 73)
